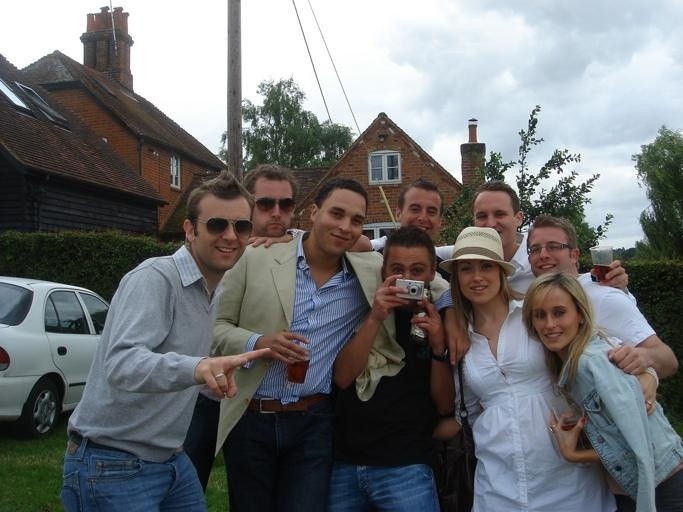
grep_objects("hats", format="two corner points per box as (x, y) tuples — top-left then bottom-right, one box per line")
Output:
(438, 226), (516, 278)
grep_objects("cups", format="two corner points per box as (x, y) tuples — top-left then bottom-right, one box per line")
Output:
(286, 345), (311, 384)
(589, 245), (615, 283)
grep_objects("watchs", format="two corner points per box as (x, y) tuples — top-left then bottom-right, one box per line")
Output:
(430, 348), (450, 362)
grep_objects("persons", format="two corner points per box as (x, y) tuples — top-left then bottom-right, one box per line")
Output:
(61, 170), (271, 511)
(434, 180), (637, 306)
(440, 227), (659, 512)
(212, 177), (471, 511)
(519, 271), (683, 511)
(526, 214), (680, 378)
(183, 163), (373, 497)
(327, 225), (457, 511)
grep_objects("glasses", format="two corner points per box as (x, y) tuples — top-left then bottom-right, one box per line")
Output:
(256, 196), (296, 214)
(198, 217), (254, 234)
(527, 241), (572, 254)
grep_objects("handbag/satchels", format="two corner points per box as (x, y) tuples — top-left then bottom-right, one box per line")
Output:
(429, 356), (476, 512)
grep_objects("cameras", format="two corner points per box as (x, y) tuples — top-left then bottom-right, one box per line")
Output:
(395, 279), (424, 300)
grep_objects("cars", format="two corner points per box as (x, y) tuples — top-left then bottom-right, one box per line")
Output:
(0, 275), (116, 434)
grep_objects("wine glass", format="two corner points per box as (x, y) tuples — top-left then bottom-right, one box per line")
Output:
(551, 393), (595, 467)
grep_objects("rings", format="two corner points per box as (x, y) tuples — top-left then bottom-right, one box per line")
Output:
(215, 374), (224, 378)
(547, 424), (557, 431)
(646, 400), (653, 407)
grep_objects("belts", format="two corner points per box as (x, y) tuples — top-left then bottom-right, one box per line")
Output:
(70, 428), (110, 452)
(247, 391), (332, 415)
